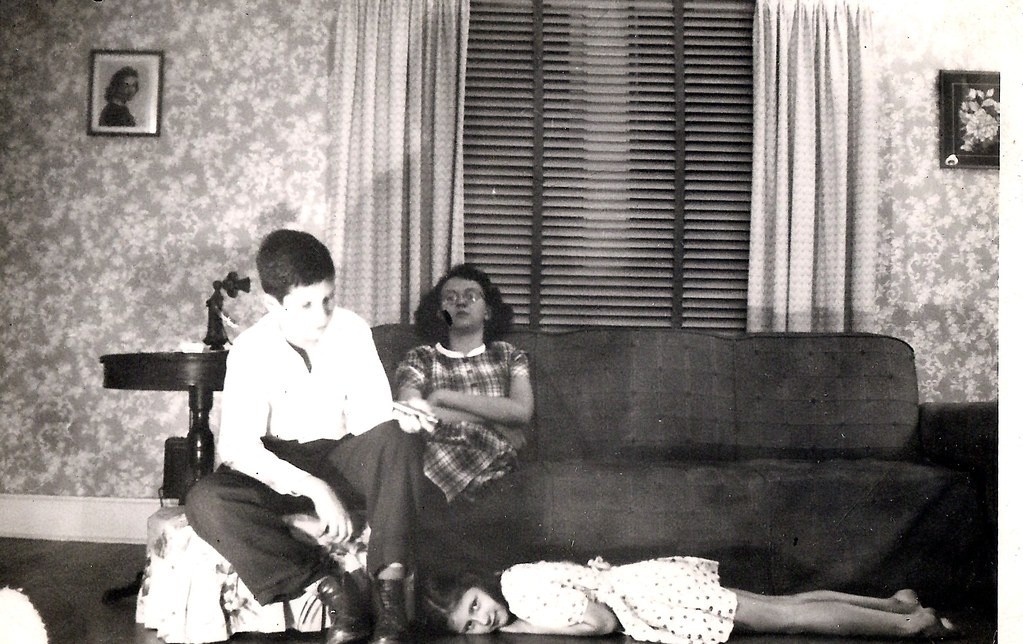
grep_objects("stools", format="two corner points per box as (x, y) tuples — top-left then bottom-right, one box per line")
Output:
(130, 507), (415, 642)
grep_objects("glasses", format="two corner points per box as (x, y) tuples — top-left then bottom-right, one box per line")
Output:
(438, 287), (488, 312)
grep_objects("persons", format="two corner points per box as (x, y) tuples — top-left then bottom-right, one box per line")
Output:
(416, 555), (968, 644)
(185, 229), (535, 644)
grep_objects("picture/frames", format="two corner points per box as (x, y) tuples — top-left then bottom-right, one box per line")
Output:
(938, 69), (1000, 171)
(86, 48), (165, 137)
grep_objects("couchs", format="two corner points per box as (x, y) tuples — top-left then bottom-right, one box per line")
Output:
(370, 328), (998, 614)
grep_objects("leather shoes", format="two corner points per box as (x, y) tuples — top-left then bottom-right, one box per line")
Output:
(315, 572), (362, 644)
(369, 578), (406, 644)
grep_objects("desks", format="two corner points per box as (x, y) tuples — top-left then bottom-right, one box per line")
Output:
(100, 351), (228, 606)
